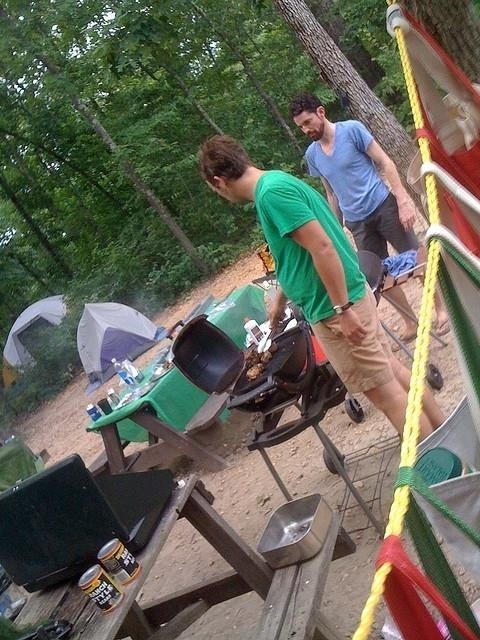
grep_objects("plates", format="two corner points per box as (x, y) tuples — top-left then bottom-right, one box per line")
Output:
(149, 363), (176, 383)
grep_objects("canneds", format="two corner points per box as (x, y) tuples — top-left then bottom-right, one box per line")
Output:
(78, 565), (125, 614)
(97, 538), (142, 586)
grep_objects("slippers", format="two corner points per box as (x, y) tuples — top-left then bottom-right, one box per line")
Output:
(390, 333), (417, 352)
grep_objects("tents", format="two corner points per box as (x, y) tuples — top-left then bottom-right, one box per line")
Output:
(0, 292), (76, 397)
(73, 299), (172, 397)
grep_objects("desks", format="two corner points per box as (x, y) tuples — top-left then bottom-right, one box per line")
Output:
(0, 470), (358, 639)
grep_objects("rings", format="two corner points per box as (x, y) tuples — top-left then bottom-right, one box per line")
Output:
(359, 333), (364, 339)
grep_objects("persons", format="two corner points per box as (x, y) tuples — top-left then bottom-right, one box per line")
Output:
(289, 90), (453, 343)
(199, 134), (447, 446)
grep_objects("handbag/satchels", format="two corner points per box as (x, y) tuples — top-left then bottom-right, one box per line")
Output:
(407, 82), (480, 580)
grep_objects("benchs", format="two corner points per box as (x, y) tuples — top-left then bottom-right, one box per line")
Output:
(83, 438), (131, 477)
(177, 348), (255, 446)
(253, 509), (342, 640)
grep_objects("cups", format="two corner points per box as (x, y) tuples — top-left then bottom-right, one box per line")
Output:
(86, 404), (102, 421)
(97, 398), (113, 415)
(107, 389), (122, 406)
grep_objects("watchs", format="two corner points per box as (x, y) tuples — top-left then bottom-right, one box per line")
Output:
(332, 301), (354, 315)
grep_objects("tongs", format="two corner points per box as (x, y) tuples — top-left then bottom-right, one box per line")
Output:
(258, 323), (277, 354)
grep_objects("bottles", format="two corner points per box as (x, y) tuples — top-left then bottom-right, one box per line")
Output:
(243, 317), (265, 346)
(111, 358), (138, 390)
